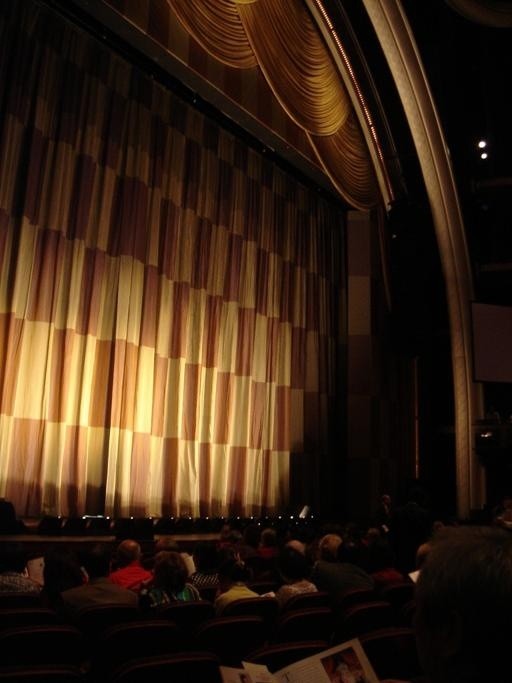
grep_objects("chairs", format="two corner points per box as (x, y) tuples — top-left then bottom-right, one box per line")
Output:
(341, 624), (425, 682)
(3, 661), (101, 682)
(268, 607), (332, 646)
(111, 649), (221, 680)
(240, 640), (327, 682)
(224, 596), (280, 639)
(146, 602), (216, 630)
(285, 589), (331, 613)
(103, 617), (179, 649)
(337, 601), (397, 644)
(67, 602), (143, 636)
(4, 590), (46, 608)
(334, 587), (378, 607)
(2, 623), (84, 661)
(3, 604), (68, 624)
(190, 614), (264, 664)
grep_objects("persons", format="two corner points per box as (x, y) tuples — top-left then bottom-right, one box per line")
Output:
(387, 497), (512, 585)
(385, 519), (510, 682)
(1, 517), (220, 612)
(213, 520), (397, 610)
(380, 494), (393, 518)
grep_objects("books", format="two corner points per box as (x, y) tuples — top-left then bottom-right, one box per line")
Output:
(219, 640), (379, 682)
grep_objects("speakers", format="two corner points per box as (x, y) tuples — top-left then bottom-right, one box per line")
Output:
(36, 514), (319, 536)
